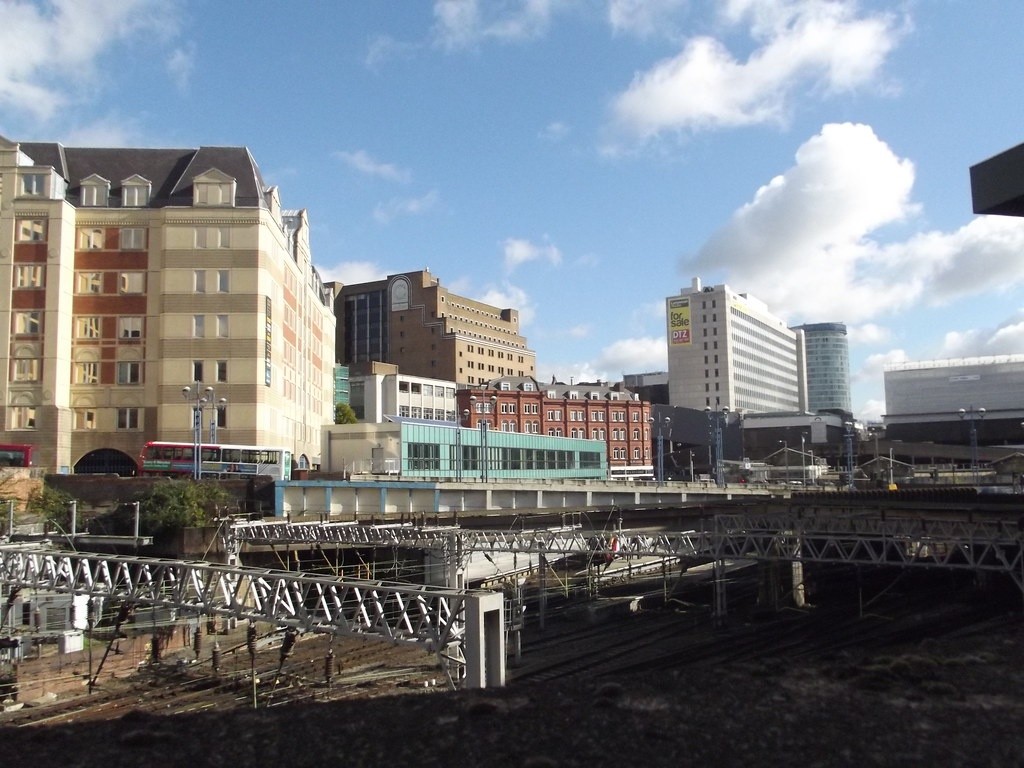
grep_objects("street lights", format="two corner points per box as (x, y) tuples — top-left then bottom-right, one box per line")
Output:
(778, 440), (790, 483)
(7, 499), (13, 537)
(199, 389), (226, 444)
(704, 400), (730, 488)
(69, 500), (77, 536)
(132, 501), (140, 538)
(842, 421), (856, 488)
(808, 450), (813, 465)
(957, 404), (986, 486)
(180, 379), (213, 481)
(469, 389), (497, 482)
(454, 403), (470, 483)
(647, 411), (671, 482)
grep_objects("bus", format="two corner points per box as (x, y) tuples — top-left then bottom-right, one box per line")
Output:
(611, 464), (655, 480)
(0, 443), (33, 467)
(137, 442), (291, 481)
